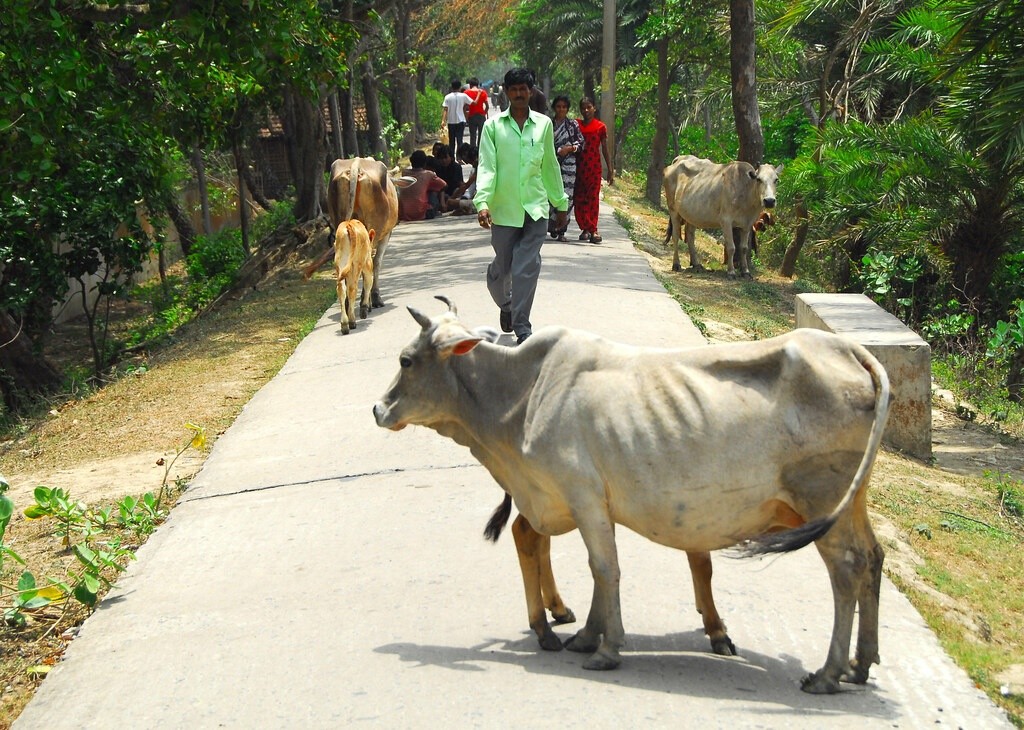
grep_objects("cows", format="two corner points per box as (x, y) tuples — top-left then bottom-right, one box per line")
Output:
(372, 296), (893, 694)
(662, 155), (784, 279)
(326, 156), (417, 335)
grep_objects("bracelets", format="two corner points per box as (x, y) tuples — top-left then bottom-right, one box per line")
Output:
(572, 145), (576, 151)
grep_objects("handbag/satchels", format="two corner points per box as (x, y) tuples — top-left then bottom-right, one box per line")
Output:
(437, 126), (449, 145)
(574, 150), (582, 163)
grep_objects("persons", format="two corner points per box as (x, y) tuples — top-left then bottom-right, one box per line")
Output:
(473, 67), (569, 345)
(399, 78), (613, 244)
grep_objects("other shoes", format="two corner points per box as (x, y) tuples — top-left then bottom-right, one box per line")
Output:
(517, 333), (532, 344)
(551, 226), (559, 238)
(462, 205), (472, 215)
(578, 232), (590, 240)
(449, 208), (463, 215)
(558, 236), (568, 242)
(500, 310), (514, 333)
(590, 236), (603, 243)
